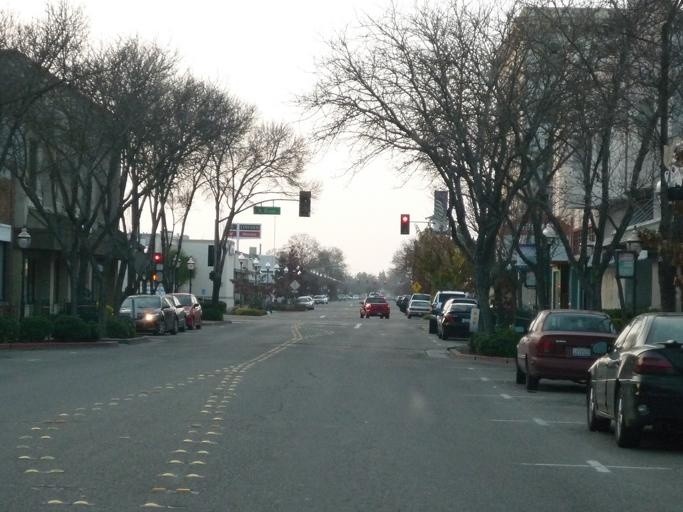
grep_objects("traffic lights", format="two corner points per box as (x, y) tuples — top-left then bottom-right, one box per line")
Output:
(149, 252), (165, 285)
(400, 213), (410, 235)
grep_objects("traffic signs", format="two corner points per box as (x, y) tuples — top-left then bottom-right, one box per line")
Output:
(253, 205), (282, 216)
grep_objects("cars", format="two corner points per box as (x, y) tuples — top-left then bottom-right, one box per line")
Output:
(396, 292), (433, 321)
(357, 296), (391, 320)
(513, 308), (619, 392)
(428, 289), (489, 340)
(295, 296), (315, 310)
(119, 291), (205, 335)
(585, 311), (683, 448)
(337, 291), (387, 302)
(313, 295), (329, 305)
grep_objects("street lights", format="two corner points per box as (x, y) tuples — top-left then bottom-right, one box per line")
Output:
(264, 261), (271, 295)
(291, 265), (346, 295)
(184, 257), (195, 295)
(251, 256), (259, 306)
(14, 224), (31, 340)
(273, 263), (279, 290)
(236, 252), (243, 308)
(653, 177), (681, 306)
(541, 222), (557, 314)
(283, 265), (288, 279)
